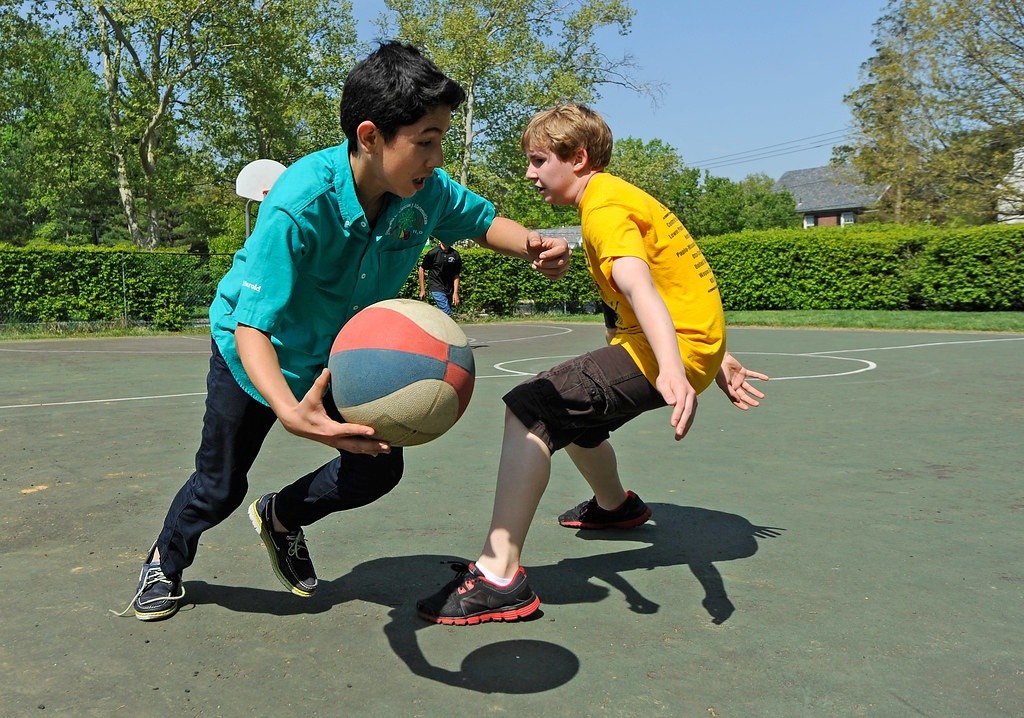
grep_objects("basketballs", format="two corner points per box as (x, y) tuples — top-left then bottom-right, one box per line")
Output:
(326, 298), (477, 451)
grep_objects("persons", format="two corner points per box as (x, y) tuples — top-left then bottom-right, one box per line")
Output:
(418, 239), (463, 320)
(415, 103), (769, 626)
(107, 39), (573, 621)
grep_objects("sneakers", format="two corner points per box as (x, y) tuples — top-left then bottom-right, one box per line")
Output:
(109, 539), (186, 621)
(558, 490), (652, 529)
(416, 557), (541, 626)
(248, 492), (318, 598)
